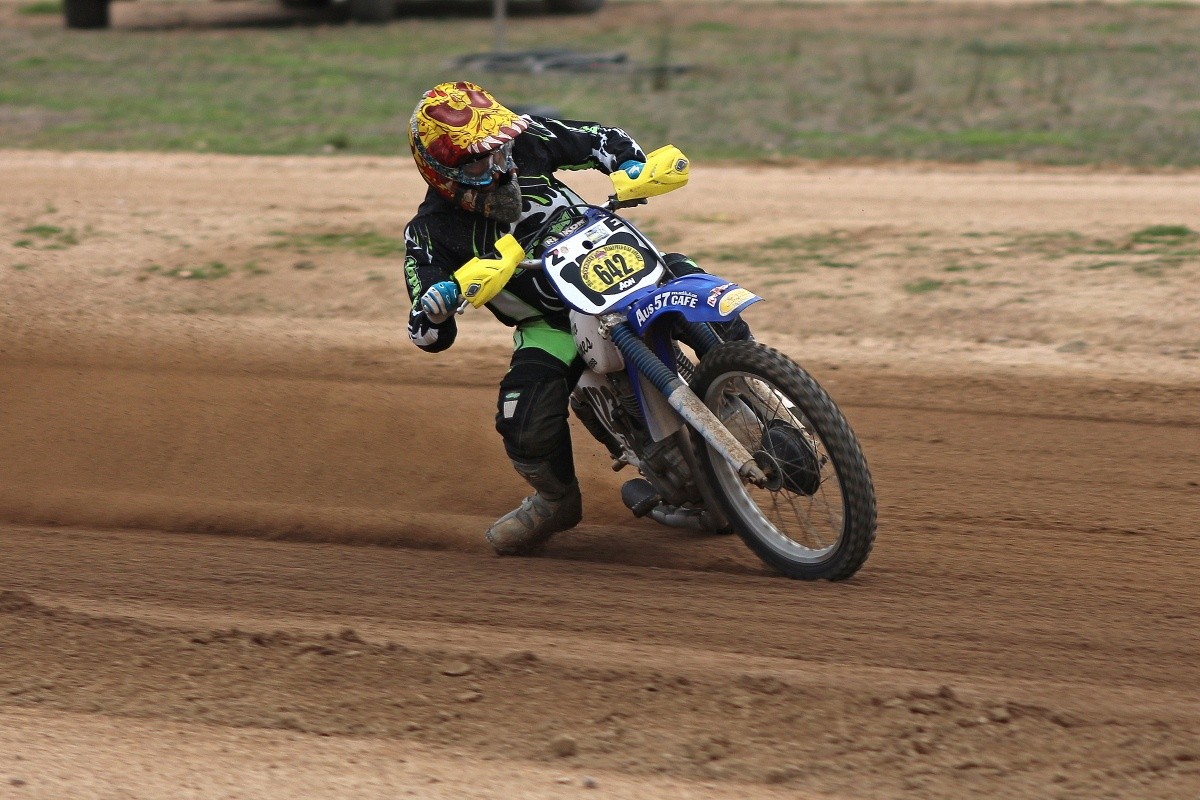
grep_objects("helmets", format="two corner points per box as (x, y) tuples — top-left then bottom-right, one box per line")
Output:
(408, 81), (529, 223)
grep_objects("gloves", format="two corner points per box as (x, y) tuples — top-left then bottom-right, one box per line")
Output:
(618, 160), (646, 179)
(421, 281), (459, 324)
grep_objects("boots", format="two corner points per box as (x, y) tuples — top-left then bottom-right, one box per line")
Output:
(486, 435), (582, 553)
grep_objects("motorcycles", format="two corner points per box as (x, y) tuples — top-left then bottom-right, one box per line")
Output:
(404, 140), (876, 584)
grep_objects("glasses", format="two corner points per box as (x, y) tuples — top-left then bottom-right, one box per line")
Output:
(459, 144), (512, 186)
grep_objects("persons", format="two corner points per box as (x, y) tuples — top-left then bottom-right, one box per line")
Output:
(403, 81), (816, 551)
